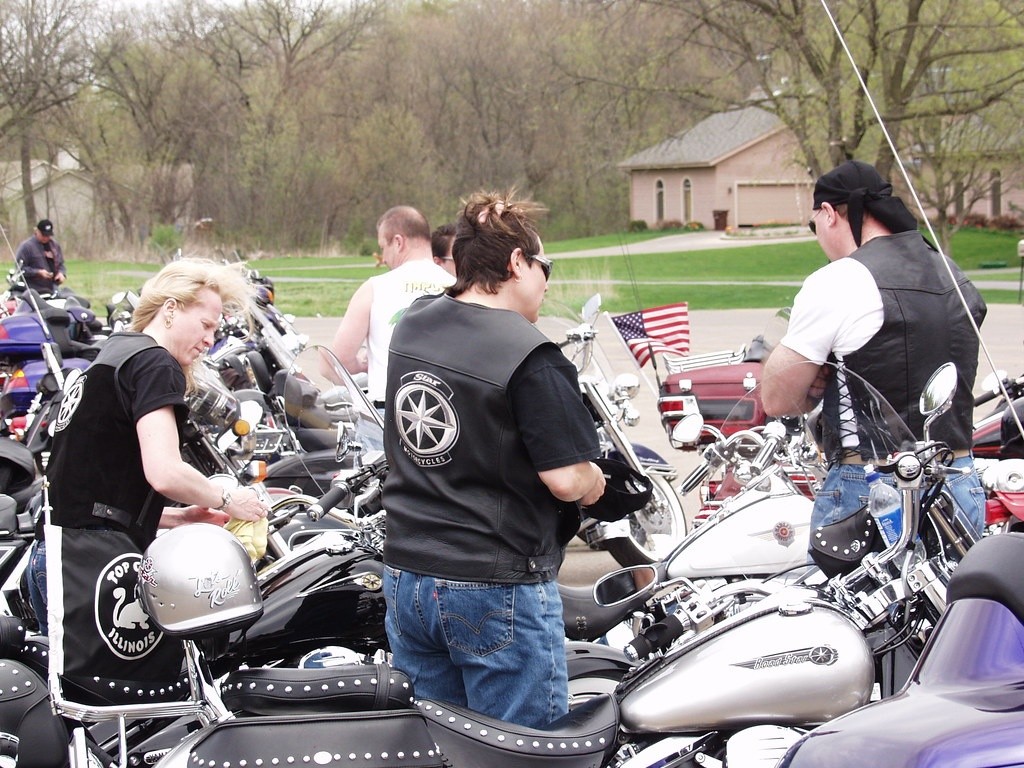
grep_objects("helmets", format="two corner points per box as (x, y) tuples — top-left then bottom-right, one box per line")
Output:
(999, 397), (1024, 454)
(137, 522), (264, 636)
(252, 277), (274, 304)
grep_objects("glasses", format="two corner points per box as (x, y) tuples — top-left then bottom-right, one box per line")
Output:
(809, 206), (837, 234)
(532, 255), (554, 282)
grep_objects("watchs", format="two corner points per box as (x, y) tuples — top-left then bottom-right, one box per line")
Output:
(216, 486), (233, 511)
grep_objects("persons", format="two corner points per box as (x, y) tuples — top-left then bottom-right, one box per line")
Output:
(761, 160), (986, 556)
(16, 219), (67, 294)
(24, 179), (605, 730)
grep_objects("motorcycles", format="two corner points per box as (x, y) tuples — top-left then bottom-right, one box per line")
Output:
(0, 283), (1024, 768)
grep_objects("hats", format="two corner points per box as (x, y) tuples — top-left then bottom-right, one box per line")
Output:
(38, 220), (53, 235)
(581, 458), (653, 523)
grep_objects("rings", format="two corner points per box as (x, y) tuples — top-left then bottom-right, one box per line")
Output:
(257, 508), (266, 516)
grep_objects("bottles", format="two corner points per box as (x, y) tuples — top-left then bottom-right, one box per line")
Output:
(863, 464), (926, 573)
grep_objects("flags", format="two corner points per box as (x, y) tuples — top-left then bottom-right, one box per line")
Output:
(611, 301), (690, 370)
